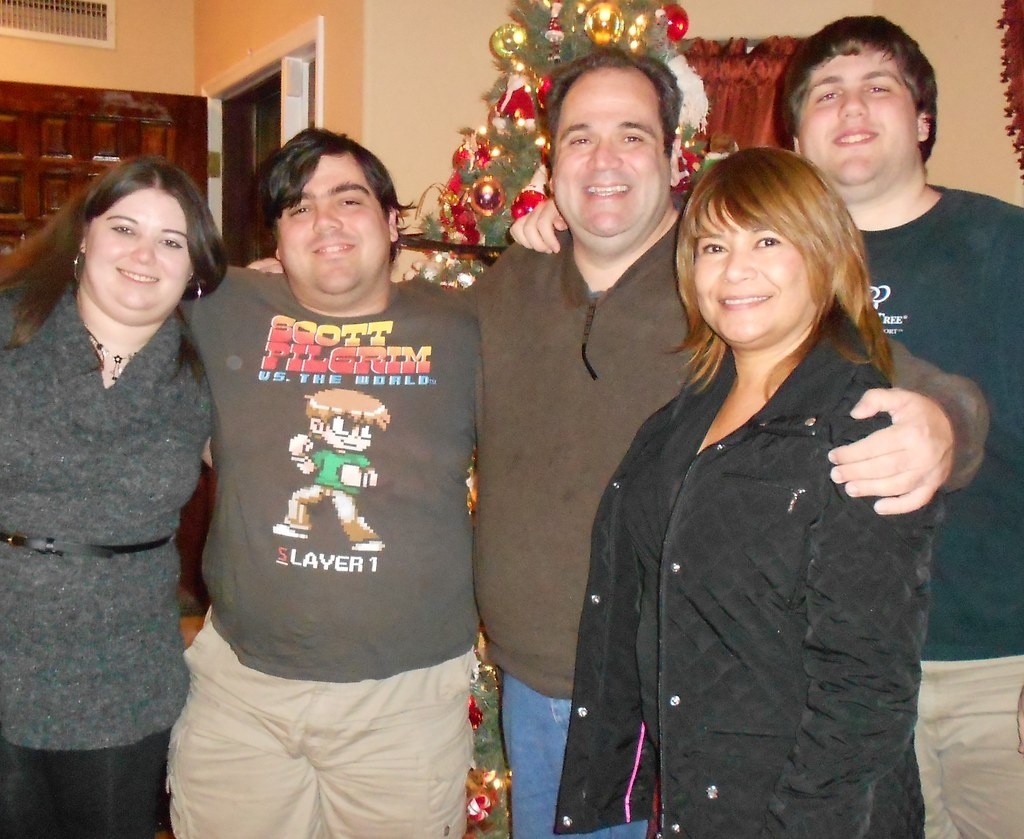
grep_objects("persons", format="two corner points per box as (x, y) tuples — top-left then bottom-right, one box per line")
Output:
(506, 12), (1024, 839)
(240, 45), (991, 839)
(165, 126), (488, 839)
(551, 142), (949, 839)
(0, 158), (227, 839)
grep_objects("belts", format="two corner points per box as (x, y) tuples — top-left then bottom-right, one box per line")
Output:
(0, 532), (174, 558)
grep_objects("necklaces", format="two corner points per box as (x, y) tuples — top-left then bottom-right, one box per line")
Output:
(83, 321), (142, 384)
